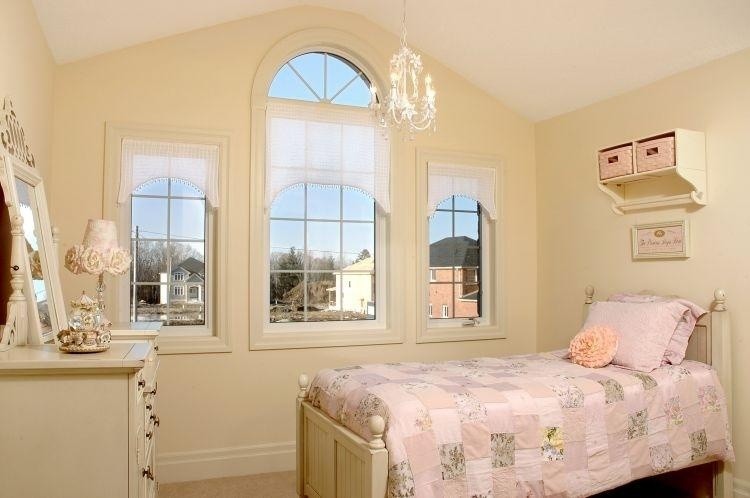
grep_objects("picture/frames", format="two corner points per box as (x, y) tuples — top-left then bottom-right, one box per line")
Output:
(631, 220), (690, 260)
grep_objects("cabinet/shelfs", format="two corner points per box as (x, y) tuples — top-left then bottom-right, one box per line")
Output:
(0, 321), (164, 498)
(595, 127), (707, 215)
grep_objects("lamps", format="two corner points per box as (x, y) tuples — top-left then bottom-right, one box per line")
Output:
(65, 218), (132, 316)
(369, 0), (438, 144)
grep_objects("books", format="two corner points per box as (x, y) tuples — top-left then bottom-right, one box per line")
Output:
(110, 329), (159, 341)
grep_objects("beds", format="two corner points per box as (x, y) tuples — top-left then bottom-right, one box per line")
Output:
(294, 285), (736, 498)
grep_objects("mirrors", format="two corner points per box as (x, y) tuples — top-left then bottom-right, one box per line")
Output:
(5, 153), (68, 345)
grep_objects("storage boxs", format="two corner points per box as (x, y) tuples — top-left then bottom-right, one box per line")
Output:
(599, 146), (633, 180)
(637, 137), (676, 172)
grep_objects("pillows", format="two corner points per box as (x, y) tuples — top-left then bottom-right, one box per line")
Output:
(609, 294), (707, 365)
(583, 301), (690, 372)
